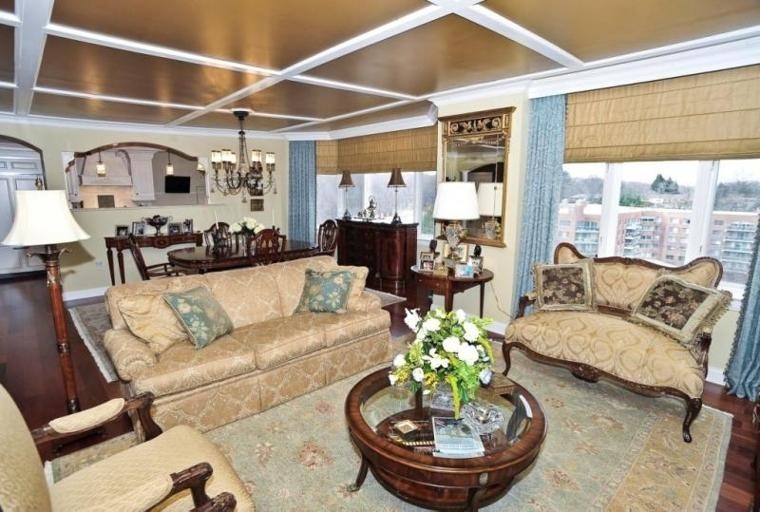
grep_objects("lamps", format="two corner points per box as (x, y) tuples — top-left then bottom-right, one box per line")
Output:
(387, 168), (407, 223)
(0, 176), (110, 455)
(336, 168), (357, 218)
(197, 110), (278, 204)
(477, 181), (503, 239)
(94, 150), (106, 175)
(430, 181), (480, 260)
(165, 151), (174, 176)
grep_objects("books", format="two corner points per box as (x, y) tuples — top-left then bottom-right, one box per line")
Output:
(391, 416), (485, 459)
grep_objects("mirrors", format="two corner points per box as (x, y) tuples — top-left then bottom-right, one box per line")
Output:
(438, 105), (516, 250)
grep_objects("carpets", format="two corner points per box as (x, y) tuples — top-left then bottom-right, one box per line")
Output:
(39, 338), (735, 512)
(66, 301), (120, 384)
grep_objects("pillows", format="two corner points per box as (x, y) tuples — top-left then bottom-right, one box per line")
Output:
(329, 263), (370, 312)
(115, 293), (189, 356)
(162, 285), (234, 350)
(529, 256), (598, 313)
(305, 265), (354, 313)
(623, 267), (732, 349)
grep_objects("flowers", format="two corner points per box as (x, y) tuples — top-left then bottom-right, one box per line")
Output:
(388, 305), (495, 421)
(228, 217), (267, 234)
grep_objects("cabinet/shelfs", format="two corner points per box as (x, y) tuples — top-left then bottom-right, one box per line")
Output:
(337, 218), (419, 338)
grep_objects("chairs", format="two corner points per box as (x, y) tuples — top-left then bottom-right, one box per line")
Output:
(307, 218), (339, 258)
(128, 234), (189, 281)
(205, 222), (234, 244)
(244, 226), (287, 266)
(0, 380), (258, 512)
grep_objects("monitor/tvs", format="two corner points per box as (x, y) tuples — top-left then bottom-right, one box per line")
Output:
(165, 175), (191, 193)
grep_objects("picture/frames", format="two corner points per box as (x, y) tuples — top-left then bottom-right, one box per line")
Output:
(171, 223), (181, 232)
(471, 256), (483, 272)
(115, 225), (128, 234)
(445, 242), (469, 261)
(132, 222), (144, 234)
(421, 251), (433, 261)
(421, 260), (434, 270)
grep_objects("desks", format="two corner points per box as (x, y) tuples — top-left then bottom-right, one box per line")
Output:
(411, 262), (493, 318)
(167, 239), (320, 276)
(104, 231), (203, 286)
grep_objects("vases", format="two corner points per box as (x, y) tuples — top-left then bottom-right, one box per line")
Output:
(242, 234), (255, 250)
(428, 381), (464, 412)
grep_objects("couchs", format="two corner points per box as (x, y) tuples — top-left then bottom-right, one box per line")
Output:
(98, 252), (395, 435)
(503, 241), (732, 444)
